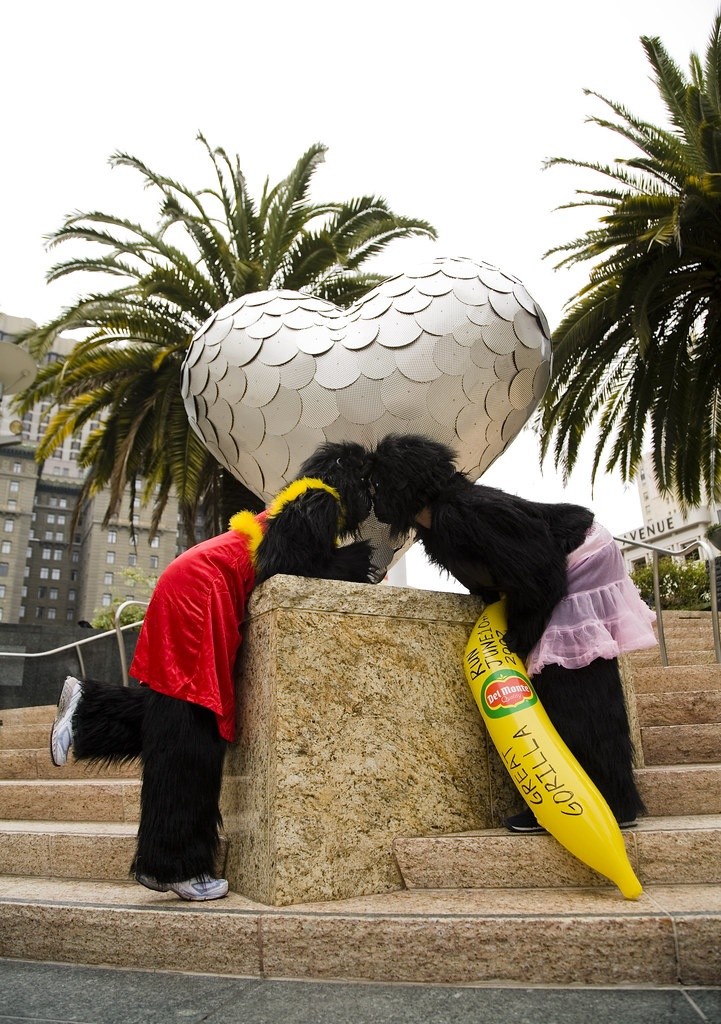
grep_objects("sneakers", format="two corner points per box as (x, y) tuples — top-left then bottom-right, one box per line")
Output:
(135, 870), (228, 901)
(50, 676), (86, 766)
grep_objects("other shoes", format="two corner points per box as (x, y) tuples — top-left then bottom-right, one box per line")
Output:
(616, 813), (638, 828)
(505, 807), (545, 831)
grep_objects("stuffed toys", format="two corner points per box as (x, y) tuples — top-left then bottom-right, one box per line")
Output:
(50, 432), (382, 902)
(369, 433), (661, 833)
